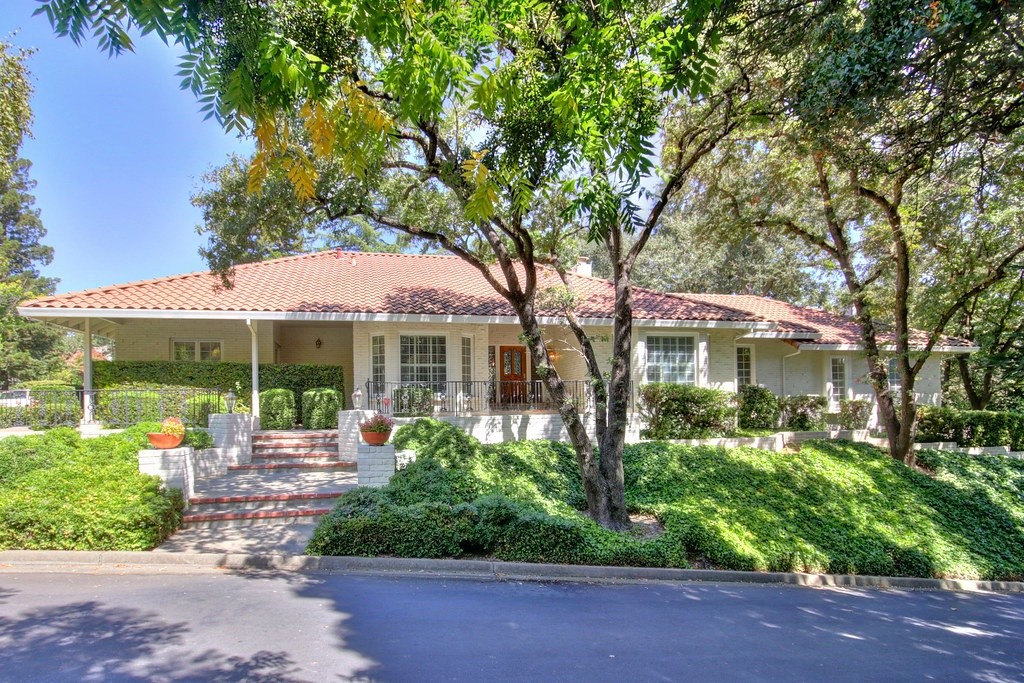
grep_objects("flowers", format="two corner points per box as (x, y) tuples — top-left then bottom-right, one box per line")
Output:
(357, 412), (394, 433)
(159, 416), (187, 436)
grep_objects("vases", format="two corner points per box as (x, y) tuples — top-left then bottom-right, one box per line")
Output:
(360, 430), (393, 446)
(146, 431), (187, 449)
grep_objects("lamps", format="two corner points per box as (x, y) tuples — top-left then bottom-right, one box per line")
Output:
(223, 388), (238, 413)
(351, 387), (365, 411)
